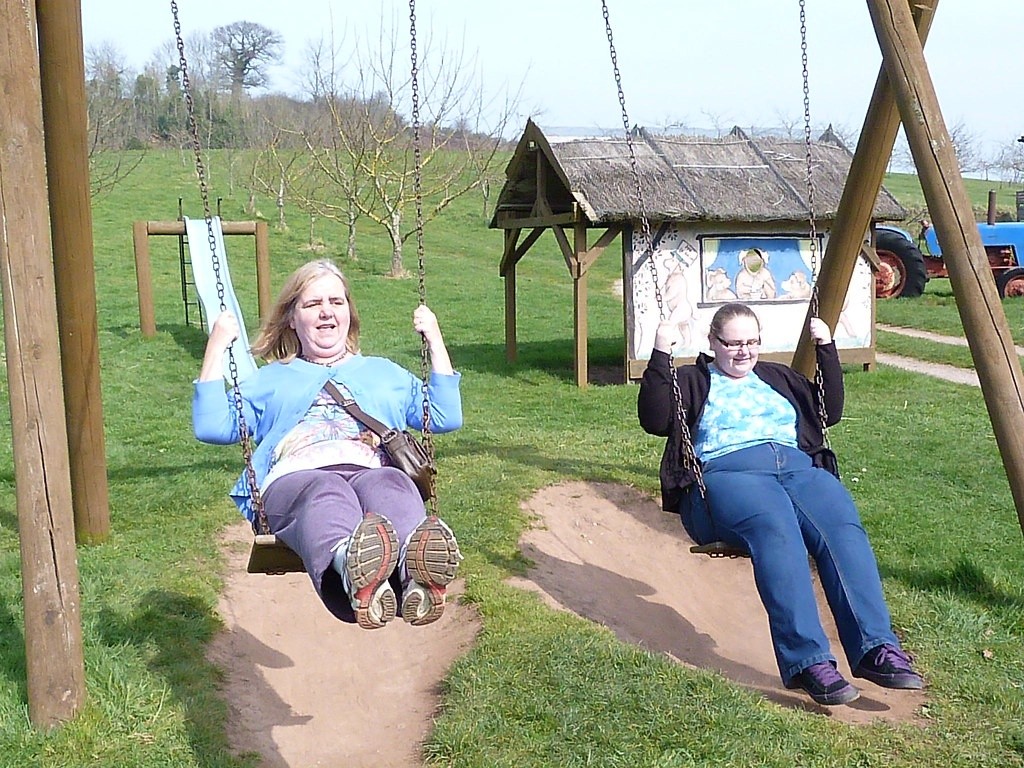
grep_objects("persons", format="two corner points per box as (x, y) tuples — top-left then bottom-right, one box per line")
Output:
(636, 303), (921, 706)
(192, 259), (463, 630)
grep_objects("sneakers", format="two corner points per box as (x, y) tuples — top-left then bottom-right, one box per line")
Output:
(853, 643), (923, 689)
(793, 660), (860, 705)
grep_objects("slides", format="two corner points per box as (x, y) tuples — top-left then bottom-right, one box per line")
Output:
(180, 214), (259, 382)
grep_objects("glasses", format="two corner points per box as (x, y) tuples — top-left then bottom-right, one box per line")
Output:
(715, 335), (763, 350)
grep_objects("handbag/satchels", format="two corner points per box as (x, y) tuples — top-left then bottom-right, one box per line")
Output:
(380, 428), (431, 503)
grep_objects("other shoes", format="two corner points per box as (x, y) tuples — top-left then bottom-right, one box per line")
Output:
(330, 511), (401, 629)
(397, 516), (464, 626)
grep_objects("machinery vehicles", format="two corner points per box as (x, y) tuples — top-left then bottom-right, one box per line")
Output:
(871, 186), (1023, 300)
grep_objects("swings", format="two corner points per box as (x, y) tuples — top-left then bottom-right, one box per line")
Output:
(603, 0), (843, 559)
(172, 0), (439, 577)
